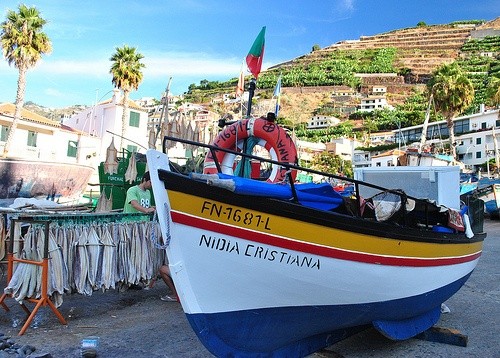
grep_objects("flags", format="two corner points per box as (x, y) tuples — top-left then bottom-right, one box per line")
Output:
(246, 27), (265, 81)
(236, 62), (245, 98)
(273, 76), (280, 116)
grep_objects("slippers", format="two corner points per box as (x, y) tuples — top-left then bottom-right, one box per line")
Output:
(161, 295), (178, 302)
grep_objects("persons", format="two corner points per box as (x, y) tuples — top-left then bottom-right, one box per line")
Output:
(152, 264), (178, 302)
(124, 171), (156, 220)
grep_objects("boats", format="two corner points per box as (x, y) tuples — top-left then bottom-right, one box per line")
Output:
(145, 77), (500, 358)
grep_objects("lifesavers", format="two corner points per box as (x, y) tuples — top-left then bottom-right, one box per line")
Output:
(203, 118), (299, 187)
